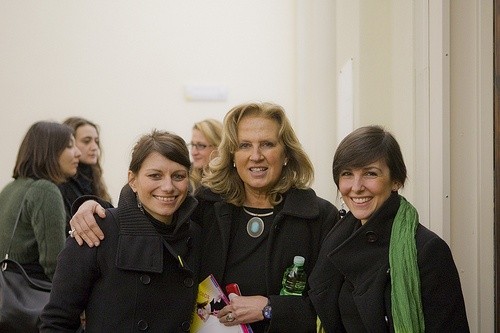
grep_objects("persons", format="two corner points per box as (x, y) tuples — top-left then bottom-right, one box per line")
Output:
(69, 102), (338, 333)
(188, 118), (225, 195)
(59, 118), (111, 204)
(308, 126), (472, 333)
(0, 122), (80, 279)
(37, 131), (202, 333)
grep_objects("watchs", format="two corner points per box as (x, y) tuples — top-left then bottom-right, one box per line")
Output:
(263, 296), (272, 321)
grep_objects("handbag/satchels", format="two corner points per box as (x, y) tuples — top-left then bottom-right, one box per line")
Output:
(0, 260), (85, 333)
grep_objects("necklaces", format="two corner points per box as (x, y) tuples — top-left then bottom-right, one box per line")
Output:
(242, 206), (273, 238)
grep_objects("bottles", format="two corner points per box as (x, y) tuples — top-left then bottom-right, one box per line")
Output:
(280, 256), (308, 297)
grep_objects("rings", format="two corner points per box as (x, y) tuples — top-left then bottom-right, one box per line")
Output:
(226, 314), (233, 321)
(69, 229), (75, 238)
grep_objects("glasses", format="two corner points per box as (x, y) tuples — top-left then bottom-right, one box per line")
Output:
(186, 143), (216, 151)
